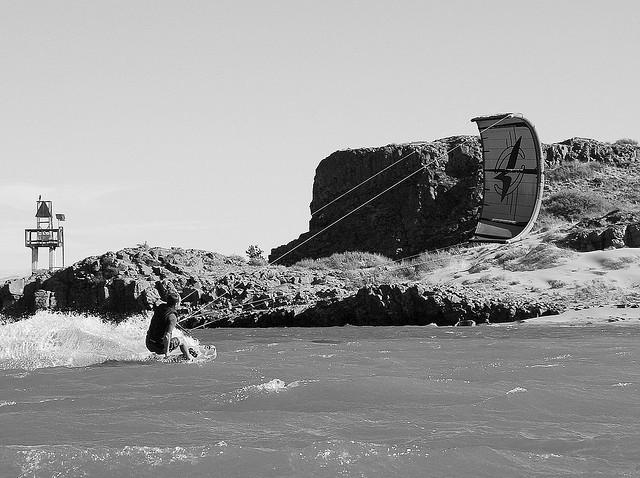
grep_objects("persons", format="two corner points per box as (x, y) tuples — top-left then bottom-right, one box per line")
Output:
(146, 293), (198, 362)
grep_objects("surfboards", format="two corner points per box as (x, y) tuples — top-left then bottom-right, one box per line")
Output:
(147, 345), (217, 364)
